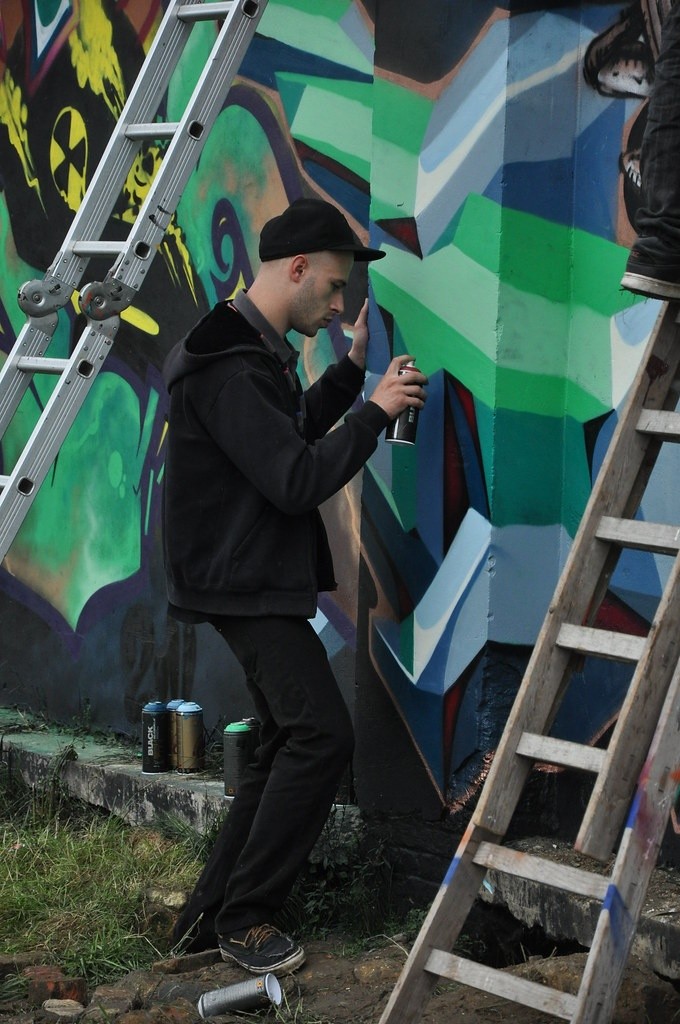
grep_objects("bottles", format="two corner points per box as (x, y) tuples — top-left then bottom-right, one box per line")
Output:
(241, 718), (261, 765)
(222, 723), (251, 798)
(385, 360), (421, 447)
(175, 702), (205, 775)
(197, 973), (282, 1018)
(141, 698), (170, 775)
(167, 698), (186, 771)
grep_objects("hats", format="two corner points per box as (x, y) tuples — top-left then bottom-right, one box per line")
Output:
(260, 198), (385, 260)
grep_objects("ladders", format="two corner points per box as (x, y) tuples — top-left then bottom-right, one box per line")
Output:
(374, 295), (680, 1024)
(0, 1), (268, 569)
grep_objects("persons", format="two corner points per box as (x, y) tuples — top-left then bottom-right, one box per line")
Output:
(154, 195), (432, 979)
(615, 1), (680, 304)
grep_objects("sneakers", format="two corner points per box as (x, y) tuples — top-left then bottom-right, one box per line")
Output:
(217, 924), (305, 976)
(617, 244), (680, 305)
